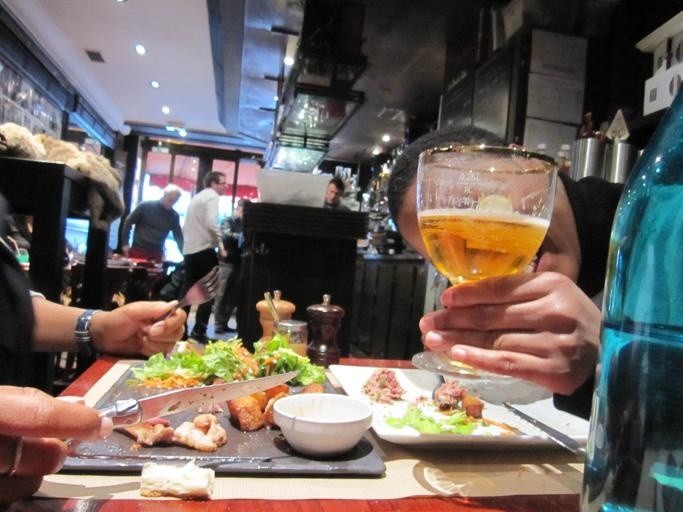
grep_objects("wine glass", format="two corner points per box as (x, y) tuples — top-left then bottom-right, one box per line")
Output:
(412, 150), (558, 379)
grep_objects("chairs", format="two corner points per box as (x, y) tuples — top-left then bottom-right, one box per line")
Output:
(62, 263), (150, 381)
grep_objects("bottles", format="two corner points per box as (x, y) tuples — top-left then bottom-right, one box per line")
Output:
(256, 290), (296, 341)
(579, 80), (683, 512)
(556, 142), (572, 173)
(535, 142), (547, 155)
(306, 295), (344, 365)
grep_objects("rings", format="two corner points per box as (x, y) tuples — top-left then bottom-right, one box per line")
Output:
(6, 436), (24, 478)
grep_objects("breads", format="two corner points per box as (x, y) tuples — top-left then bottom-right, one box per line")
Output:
(139, 459), (216, 501)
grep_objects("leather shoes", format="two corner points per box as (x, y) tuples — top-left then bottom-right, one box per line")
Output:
(190, 330), (218, 345)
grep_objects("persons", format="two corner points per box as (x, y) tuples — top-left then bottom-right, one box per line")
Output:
(121, 182), (185, 301)
(1, 194), (188, 504)
(214, 198), (252, 340)
(387, 124), (682, 450)
(323, 175), (351, 209)
(180, 171), (228, 345)
(5, 212), (73, 271)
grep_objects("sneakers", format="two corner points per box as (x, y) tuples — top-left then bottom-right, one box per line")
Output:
(215, 326), (236, 333)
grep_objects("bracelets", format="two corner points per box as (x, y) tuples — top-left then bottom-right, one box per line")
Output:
(72, 309), (101, 354)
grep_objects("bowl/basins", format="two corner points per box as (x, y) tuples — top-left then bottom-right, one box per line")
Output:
(273, 393), (372, 457)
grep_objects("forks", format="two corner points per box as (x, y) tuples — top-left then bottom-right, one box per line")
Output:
(152, 266), (223, 325)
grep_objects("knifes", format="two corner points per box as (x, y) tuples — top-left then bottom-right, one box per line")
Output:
(64, 370), (300, 450)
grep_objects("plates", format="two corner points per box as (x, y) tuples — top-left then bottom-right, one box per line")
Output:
(325, 365), (589, 445)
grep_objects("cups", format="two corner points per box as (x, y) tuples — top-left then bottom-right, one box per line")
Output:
(277, 320), (308, 358)
